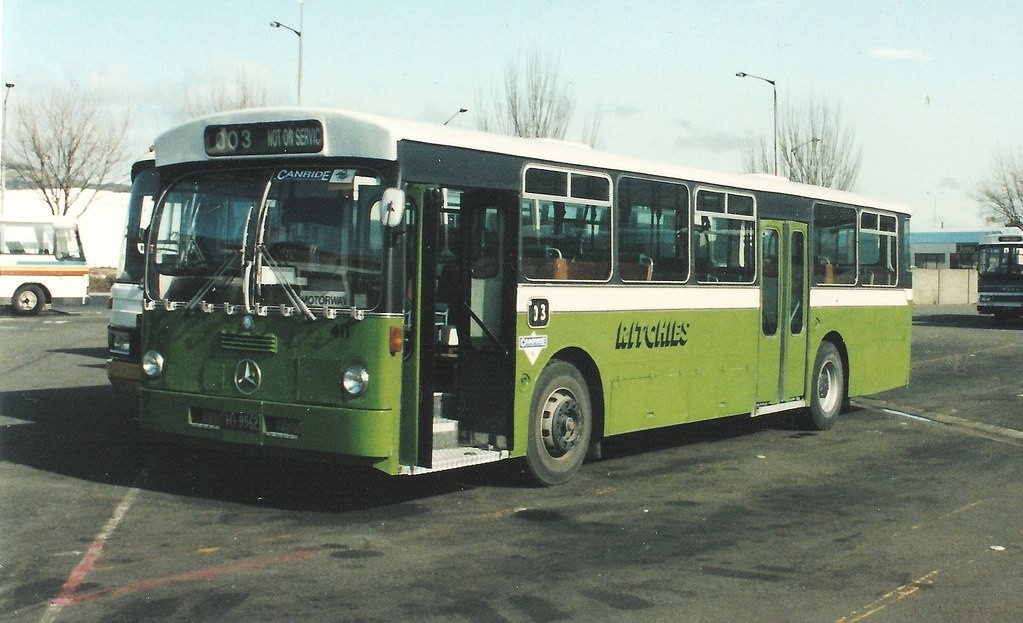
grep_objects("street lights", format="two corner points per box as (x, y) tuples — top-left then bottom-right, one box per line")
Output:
(444, 107), (468, 124)
(269, 21), (303, 104)
(0, 81), (15, 212)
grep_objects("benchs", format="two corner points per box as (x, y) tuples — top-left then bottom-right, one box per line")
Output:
(814, 257), (835, 283)
(567, 251), (653, 281)
(523, 242), (569, 279)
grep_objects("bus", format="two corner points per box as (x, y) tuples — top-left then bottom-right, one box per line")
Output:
(910, 227), (1023, 270)
(790, 137), (821, 181)
(0, 212), (89, 317)
(106, 143), (186, 414)
(735, 71), (778, 176)
(136, 103), (913, 486)
(976, 227), (1023, 321)
